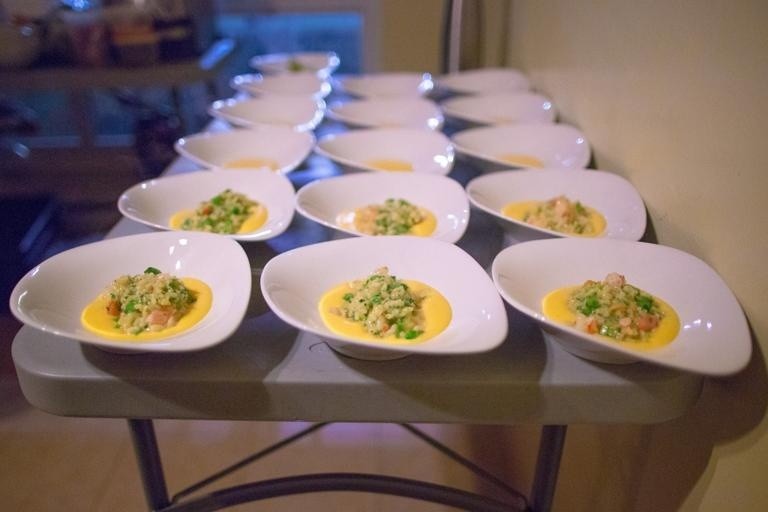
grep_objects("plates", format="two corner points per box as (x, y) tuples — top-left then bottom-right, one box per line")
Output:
(449, 121), (591, 170)
(118, 167), (295, 242)
(295, 170), (471, 244)
(260, 237), (509, 363)
(442, 94), (559, 126)
(491, 238), (752, 380)
(175, 126), (313, 175)
(328, 99), (442, 131)
(313, 129), (453, 174)
(9, 232), (252, 356)
(253, 54), (340, 74)
(436, 65), (532, 95)
(230, 74), (332, 100)
(341, 71), (433, 99)
(466, 166), (648, 244)
(208, 97), (327, 131)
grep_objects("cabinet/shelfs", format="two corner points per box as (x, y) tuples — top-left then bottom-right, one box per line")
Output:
(11, 71), (704, 512)
